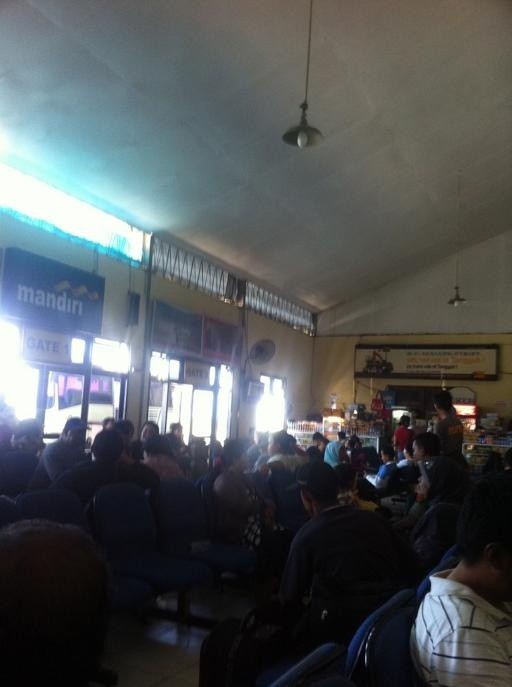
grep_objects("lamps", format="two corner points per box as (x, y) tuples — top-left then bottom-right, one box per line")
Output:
(282, 1), (326, 152)
(446, 249), (466, 309)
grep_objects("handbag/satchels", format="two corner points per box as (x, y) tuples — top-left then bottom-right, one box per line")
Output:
(239, 485), (289, 556)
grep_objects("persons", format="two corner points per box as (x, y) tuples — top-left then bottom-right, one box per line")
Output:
(1, 386), (512, 687)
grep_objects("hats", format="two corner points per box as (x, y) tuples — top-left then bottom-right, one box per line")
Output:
(65, 417), (92, 431)
